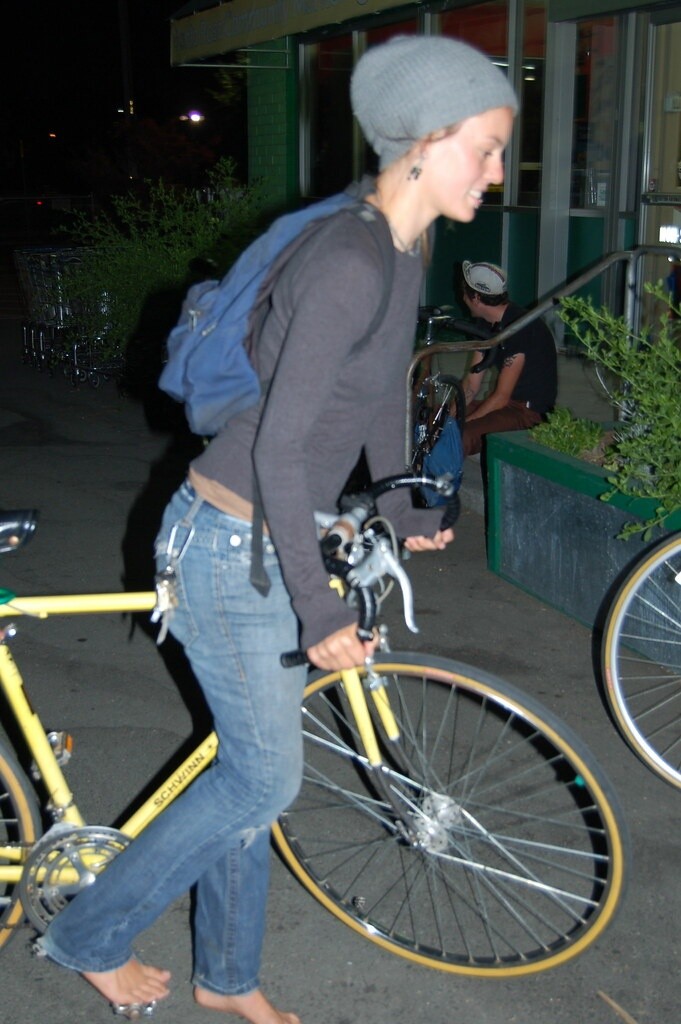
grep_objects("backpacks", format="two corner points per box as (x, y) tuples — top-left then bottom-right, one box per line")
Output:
(158, 176), (395, 435)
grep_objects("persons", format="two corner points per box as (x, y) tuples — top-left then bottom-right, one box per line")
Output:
(401, 261), (558, 463)
(34, 31), (524, 1024)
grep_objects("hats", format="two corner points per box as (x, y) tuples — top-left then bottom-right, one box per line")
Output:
(349, 35), (519, 172)
(462, 260), (507, 295)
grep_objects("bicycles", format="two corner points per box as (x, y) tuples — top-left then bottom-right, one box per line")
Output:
(410, 304), (468, 513)
(601, 529), (681, 791)
(0, 471), (628, 1018)
